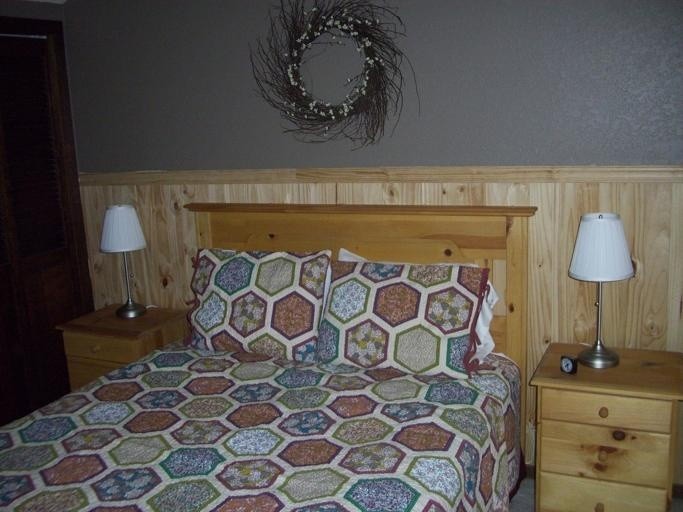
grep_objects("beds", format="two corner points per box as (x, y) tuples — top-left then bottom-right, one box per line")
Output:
(0, 200), (535, 511)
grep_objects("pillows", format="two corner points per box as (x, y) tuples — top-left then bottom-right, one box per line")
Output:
(179, 244), (331, 367)
(323, 244), (499, 364)
(313, 256), (487, 380)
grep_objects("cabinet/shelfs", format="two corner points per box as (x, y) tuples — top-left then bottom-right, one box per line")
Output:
(0, 18), (93, 430)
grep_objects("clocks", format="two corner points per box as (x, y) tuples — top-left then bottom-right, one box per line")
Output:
(558, 354), (580, 375)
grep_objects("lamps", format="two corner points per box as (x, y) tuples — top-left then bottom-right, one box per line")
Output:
(566, 213), (634, 371)
(98, 203), (150, 320)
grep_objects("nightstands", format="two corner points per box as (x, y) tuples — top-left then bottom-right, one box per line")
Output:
(55, 307), (185, 388)
(528, 341), (683, 510)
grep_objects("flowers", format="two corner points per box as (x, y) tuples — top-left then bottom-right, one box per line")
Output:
(249, 1), (424, 153)
